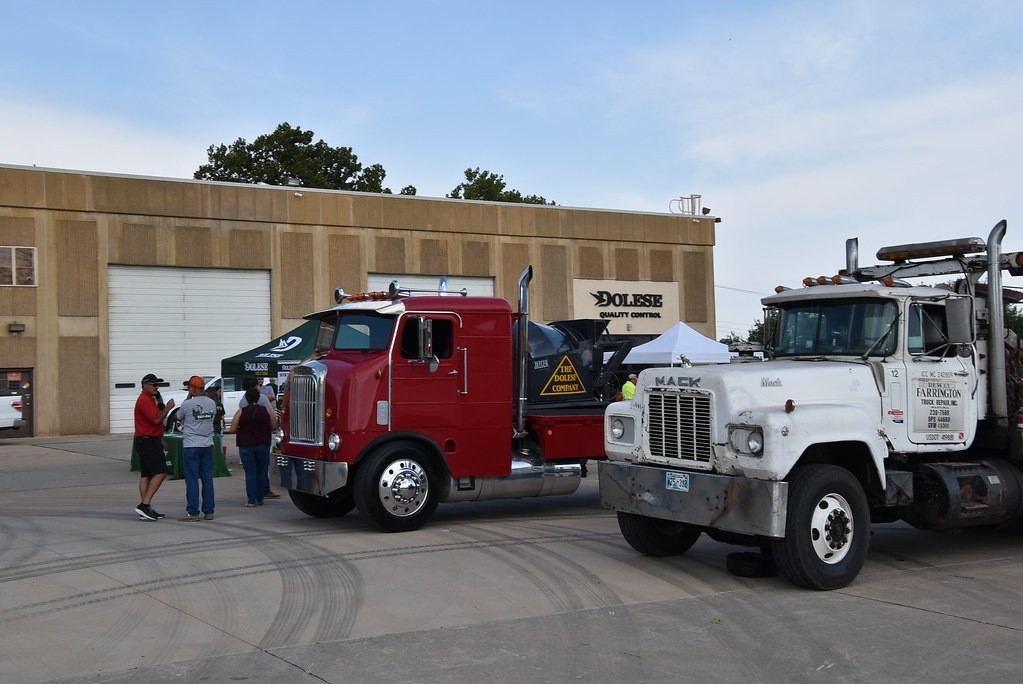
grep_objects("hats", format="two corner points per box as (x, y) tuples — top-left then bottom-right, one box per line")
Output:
(142, 374), (163, 383)
(183, 376), (204, 389)
(629, 374), (638, 379)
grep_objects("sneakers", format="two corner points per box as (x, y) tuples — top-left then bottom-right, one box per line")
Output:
(140, 512), (165, 520)
(135, 503), (157, 520)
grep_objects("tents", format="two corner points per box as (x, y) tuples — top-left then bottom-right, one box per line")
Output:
(622, 320), (730, 367)
(221, 320), (370, 437)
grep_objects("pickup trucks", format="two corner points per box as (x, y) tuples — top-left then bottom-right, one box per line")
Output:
(0, 394), (24, 432)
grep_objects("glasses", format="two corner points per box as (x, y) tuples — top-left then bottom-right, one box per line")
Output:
(256, 378), (264, 380)
(149, 383), (159, 387)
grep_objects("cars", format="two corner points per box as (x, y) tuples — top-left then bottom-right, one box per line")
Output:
(159, 374), (255, 434)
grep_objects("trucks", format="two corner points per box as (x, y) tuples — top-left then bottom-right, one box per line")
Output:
(596, 219), (1023, 592)
(273, 264), (622, 534)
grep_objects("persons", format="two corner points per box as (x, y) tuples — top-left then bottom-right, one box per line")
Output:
(621, 374), (637, 399)
(174, 376), (216, 522)
(152, 391), (165, 412)
(230, 388), (272, 508)
(204, 386), (226, 444)
(265, 378), (278, 396)
(276, 454), (308, 492)
(133, 373), (175, 521)
(238, 378), (281, 498)
(257, 377), (275, 402)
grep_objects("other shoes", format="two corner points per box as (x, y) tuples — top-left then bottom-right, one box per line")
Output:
(256, 501), (264, 505)
(228, 468), (232, 471)
(178, 513), (200, 522)
(264, 491), (280, 499)
(245, 500), (255, 507)
(204, 513), (214, 520)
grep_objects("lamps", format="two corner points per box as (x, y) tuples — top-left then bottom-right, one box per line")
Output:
(8, 323), (25, 331)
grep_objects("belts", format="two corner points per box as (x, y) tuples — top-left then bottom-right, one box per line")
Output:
(136, 436), (151, 438)
